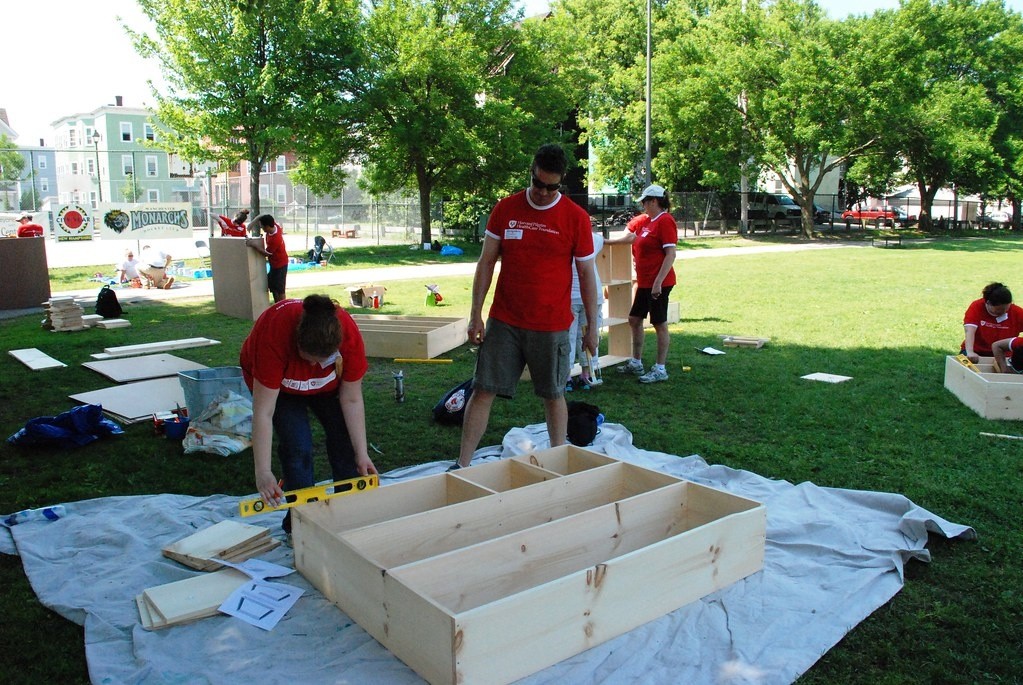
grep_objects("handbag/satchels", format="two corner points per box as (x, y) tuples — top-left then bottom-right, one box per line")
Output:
(424, 284), (439, 305)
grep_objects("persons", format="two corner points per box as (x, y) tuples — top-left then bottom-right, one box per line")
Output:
(244, 214), (289, 303)
(444, 144), (598, 472)
(992, 337), (1023, 374)
(15, 211), (43, 237)
(135, 245), (174, 289)
(566, 184), (678, 391)
(209, 210), (249, 238)
(959, 283), (1023, 364)
(239, 295), (381, 550)
(119, 249), (139, 286)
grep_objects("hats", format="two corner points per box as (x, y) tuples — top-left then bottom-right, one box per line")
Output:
(15, 211), (32, 222)
(634, 184), (665, 202)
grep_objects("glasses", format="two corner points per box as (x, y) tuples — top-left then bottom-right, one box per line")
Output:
(531, 169), (563, 191)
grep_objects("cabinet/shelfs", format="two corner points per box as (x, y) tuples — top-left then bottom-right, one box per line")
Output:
(944, 355), (1023, 421)
(351, 312), (469, 360)
(518, 240), (633, 381)
(291, 443), (767, 685)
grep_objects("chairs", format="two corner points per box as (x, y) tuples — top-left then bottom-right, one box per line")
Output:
(196, 241), (210, 269)
(313, 236), (336, 262)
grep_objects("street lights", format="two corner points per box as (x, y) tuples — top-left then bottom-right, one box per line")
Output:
(92, 130), (102, 201)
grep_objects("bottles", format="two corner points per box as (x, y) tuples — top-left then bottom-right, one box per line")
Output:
(393, 370), (404, 403)
(373, 291), (382, 307)
(368, 296), (372, 308)
(596, 413), (605, 425)
(374, 296), (379, 308)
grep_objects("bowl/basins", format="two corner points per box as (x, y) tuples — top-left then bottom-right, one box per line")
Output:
(164, 416), (189, 445)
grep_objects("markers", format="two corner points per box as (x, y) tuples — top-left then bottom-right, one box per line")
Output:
(273, 477), (284, 501)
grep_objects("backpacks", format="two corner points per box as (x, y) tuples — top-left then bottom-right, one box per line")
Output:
(95, 285), (122, 318)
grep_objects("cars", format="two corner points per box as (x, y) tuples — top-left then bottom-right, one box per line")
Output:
(885, 207), (917, 227)
(976, 211), (1013, 227)
(790, 199), (830, 225)
(842, 206), (899, 223)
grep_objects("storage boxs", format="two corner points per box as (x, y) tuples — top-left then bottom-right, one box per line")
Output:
(177, 365), (253, 420)
(346, 285), (387, 306)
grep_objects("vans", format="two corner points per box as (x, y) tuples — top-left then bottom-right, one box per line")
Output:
(736, 193), (801, 218)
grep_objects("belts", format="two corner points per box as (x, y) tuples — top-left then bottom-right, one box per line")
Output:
(149, 264), (166, 269)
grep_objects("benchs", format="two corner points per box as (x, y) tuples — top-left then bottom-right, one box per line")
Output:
(865, 235), (905, 246)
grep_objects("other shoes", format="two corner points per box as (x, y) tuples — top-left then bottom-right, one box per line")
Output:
(144, 284), (150, 289)
(163, 277), (174, 289)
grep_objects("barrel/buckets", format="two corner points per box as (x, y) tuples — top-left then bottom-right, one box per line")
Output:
(308, 262), (316, 268)
(424, 243), (431, 249)
(173, 409), (188, 418)
(320, 260), (328, 266)
(288, 258), (295, 263)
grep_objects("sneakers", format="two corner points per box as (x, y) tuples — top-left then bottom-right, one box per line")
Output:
(616, 362), (645, 375)
(638, 364), (668, 383)
(445, 462), (471, 472)
(565, 377), (573, 391)
(579, 374), (590, 391)
